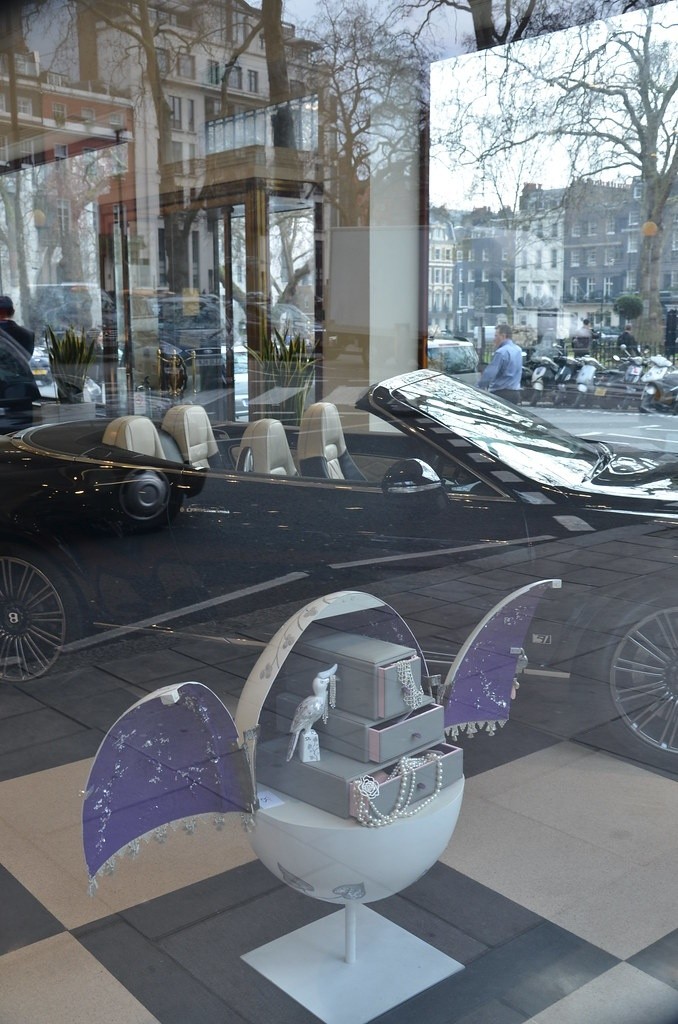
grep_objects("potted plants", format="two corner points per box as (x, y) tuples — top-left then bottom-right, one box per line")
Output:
(27, 327), (98, 428)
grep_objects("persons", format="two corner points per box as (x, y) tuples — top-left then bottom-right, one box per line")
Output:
(478, 325), (522, 402)
(0, 295), (37, 428)
(617, 324), (640, 356)
(573, 320), (600, 357)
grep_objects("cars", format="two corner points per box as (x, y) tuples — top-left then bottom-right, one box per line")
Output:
(21, 282), (315, 363)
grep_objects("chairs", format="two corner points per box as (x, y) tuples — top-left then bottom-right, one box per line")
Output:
(239, 418), (307, 476)
(296, 401), (370, 484)
(164, 404), (224, 470)
(103, 415), (167, 463)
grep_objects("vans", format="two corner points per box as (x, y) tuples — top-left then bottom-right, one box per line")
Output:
(428, 337), (484, 389)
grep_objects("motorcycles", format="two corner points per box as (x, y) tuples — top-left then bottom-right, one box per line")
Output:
(524, 353), (678, 411)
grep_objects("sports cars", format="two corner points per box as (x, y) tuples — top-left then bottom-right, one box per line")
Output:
(0, 366), (678, 752)
